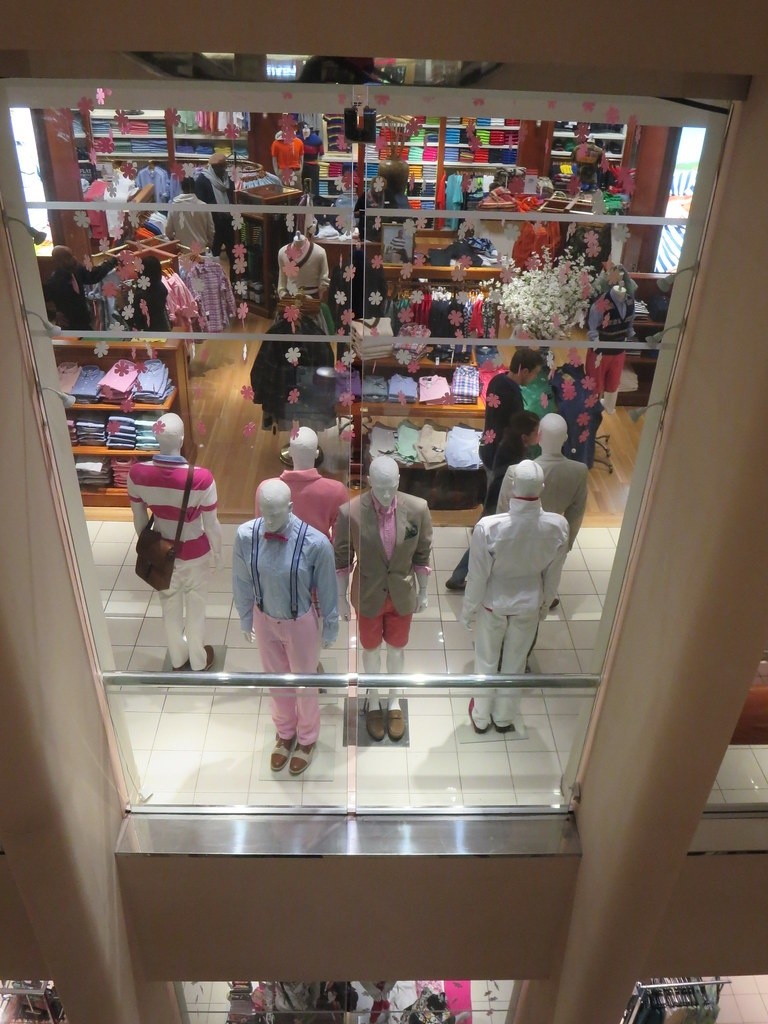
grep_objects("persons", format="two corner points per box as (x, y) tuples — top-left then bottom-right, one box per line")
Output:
(390, 230), (410, 263)
(47, 246), (134, 330)
(255, 427), (350, 637)
(359, 980), (396, 1022)
(195, 153), (235, 284)
(445, 410), (560, 610)
(334, 456), (434, 740)
(277, 230), (331, 314)
(572, 135), (603, 192)
(461, 459), (570, 733)
(119, 256), (172, 332)
(479, 347), (544, 492)
(585, 280), (636, 414)
(232, 477), (339, 774)
(272, 125), (324, 195)
(165, 177), (215, 256)
(127, 413), (224, 671)
(496, 413), (587, 672)
(354, 187), (386, 242)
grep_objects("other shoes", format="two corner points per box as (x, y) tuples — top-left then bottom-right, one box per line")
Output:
(173, 658), (190, 671)
(192, 645), (215, 671)
(445, 578), (468, 589)
(469, 697), (489, 733)
(490, 713), (512, 733)
(316, 661), (325, 692)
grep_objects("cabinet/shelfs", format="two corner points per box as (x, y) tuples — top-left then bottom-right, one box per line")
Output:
(334, 346), (487, 511)
(232, 185), (304, 318)
(69, 108), (636, 238)
(616, 273), (676, 407)
(50, 328), (197, 511)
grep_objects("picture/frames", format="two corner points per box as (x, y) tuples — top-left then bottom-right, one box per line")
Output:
(380, 223), (414, 267)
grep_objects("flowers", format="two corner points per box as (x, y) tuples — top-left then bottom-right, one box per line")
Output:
(481, 244), (608, 340)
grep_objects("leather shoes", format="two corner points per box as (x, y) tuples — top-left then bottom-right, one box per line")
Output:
(290, 743), (314, 773)
(270, 738), (293, 771)
(367, 702), (385, 741)
(387, 704), (405, 740)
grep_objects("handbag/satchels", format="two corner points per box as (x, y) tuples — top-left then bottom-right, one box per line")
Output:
(135, 529), (176, 591)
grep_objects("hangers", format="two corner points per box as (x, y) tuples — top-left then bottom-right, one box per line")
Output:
(126, 209), (153, 237)
(240, 168), (267, 182)
(159, 260), (175, 277)
(190, 249), (205, 265)
(148, 160), (155, 170)
(386, 280), (496, 310)
